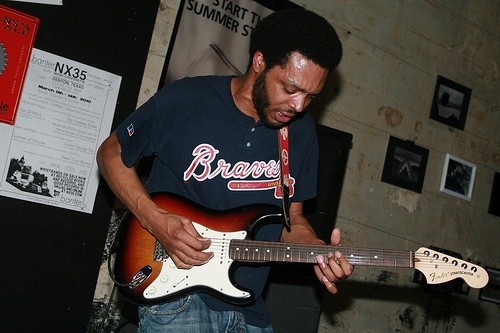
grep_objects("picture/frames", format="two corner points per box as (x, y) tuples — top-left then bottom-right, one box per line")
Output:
(429, 74), (472, 132)
(438, 153), (477, 202)
(380, 135), (429, 193)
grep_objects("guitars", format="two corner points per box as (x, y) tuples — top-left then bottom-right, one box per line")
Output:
(107, 191), (490, 308)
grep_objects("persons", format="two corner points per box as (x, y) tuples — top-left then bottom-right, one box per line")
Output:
(96, 7), (355, 333)
